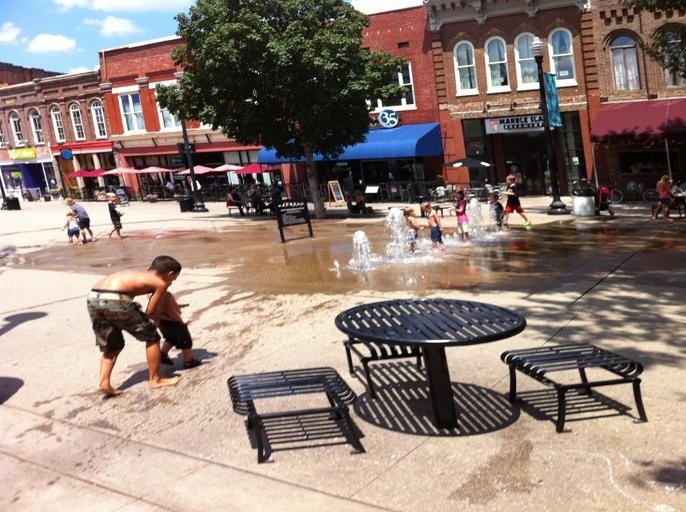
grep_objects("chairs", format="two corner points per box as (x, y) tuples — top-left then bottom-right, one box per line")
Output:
(429, 181), (456, 203)
(486, 184), (500, 196)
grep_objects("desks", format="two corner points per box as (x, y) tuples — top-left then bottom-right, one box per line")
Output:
(336, 298), (525, 429)
(464, 187), (484, 201)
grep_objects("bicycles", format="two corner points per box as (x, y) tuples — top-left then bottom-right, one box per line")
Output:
(607, 177), (658, 204)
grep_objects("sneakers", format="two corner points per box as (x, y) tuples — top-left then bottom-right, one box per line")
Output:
(502, 220), (511, 230)
(651, 215), (658, 220)
(663, 216), (676, 224)
(159, 349), (176, 366)
(523, 219), (532, 227)
(183, 358), (206, 369)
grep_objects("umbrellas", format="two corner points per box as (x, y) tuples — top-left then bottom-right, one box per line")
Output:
(84, 167), (108, 194)
(212, 164), (243, 186)
(67, 167), (90, 192)
(135, 166), (178, 199)
(177, 165), (214, 191)
(235, 163), (280, 175)
(102, 165), (139, 202)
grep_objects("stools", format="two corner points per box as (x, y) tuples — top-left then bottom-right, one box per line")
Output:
(229, 367), (365, 461)
(499, 340), (647, 433)
(344, 334), (427, 397)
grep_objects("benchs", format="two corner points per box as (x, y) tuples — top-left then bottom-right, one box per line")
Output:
(225, 196), (244, 215)
(246, 199), (283, 216)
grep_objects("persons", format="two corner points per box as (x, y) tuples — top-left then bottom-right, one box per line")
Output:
(402, 206), (423, 256)
(64, 197), (95, 241)
(245, 183), (261, 204)
(105, 191), (124, 240)
(449, 188), (470, 243)
(62, 211), (83, 246)
(149, 291), (202, 369)
(488, 192), (505, 228)
(352, 189), (363, 206)
(86, 255), (182, 394)
(227, 186), (245, 217)
(500, 175), (534, 233)
(651, 174), (675, 221)
(421, 201), (443, 255)
(263, 182), (282, 214)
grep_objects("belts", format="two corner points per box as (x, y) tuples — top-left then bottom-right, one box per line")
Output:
(87, 290), (134, 304)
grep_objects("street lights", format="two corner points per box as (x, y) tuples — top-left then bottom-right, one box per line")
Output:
(181, 118), (208, 212)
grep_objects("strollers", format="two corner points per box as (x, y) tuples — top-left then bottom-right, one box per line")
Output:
(594, 180), (615, 216)
